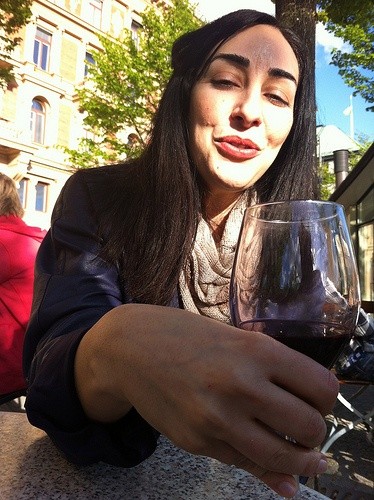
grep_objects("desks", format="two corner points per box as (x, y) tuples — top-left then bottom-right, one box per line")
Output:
(0, 411), (332, 500)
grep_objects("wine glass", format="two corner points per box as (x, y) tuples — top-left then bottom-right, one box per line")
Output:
(228, 198), (362, 500)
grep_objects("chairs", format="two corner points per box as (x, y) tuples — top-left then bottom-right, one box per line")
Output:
(313, 367), (374, 500)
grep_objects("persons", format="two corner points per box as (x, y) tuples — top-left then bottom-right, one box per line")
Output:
(0, 172), (47, 406)
(21, 9), (341, 500)
